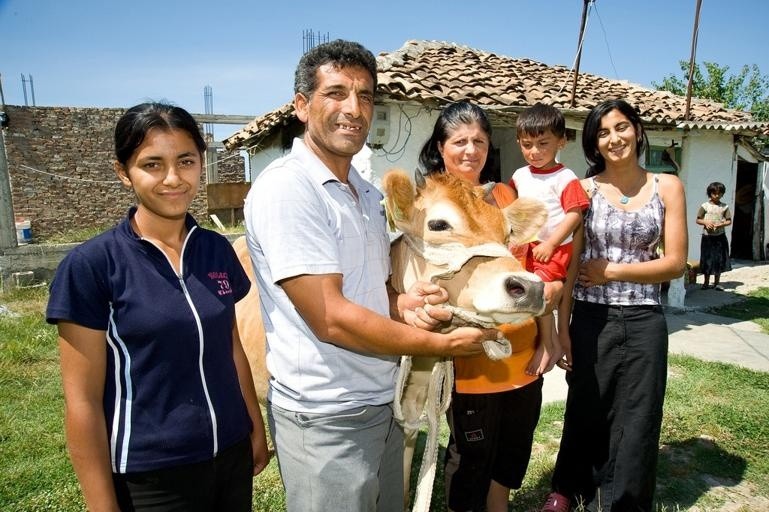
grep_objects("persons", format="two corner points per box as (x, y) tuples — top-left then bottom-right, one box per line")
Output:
(420, 99), (565, 512)
(507, 103), (592, 377)
(244, 39), (505, 512)
(696, 183), (732, 290)
(46, 96), (269, 511)
(539, 98), (688, 511)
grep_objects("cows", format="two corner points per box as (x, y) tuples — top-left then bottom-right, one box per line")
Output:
(231, 167), (549, 512)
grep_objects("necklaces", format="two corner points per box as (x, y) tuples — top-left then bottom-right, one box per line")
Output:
(607, 168), (644, 204)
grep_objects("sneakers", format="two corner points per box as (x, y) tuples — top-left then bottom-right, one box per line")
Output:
(541, 492), (571, 512)
